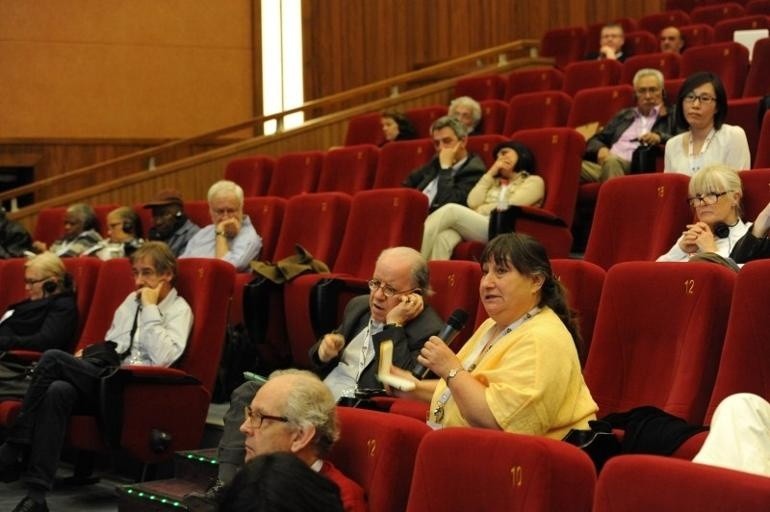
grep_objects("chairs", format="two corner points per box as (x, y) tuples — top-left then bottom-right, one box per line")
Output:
(0, 0), (770, 512)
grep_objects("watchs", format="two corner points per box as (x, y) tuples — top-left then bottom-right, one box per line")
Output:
(445, 367), (465, 385)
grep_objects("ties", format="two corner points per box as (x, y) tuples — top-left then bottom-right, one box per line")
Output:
(119, 305), (139, 359)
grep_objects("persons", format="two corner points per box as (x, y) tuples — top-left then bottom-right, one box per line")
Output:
(663, 71), (751, 175)
(178, 179), (261, 272)
(380, 98), (546, 260)
(689, 201), (770, 271)
(656, 167), (751, 262)
(385, 234), (601, 442)
(0, 242), (194, 512)
(580, 69), (690, 182)
(693, 393), (770, 475)
(1, 251), (78, 353)
(239, 369), (367, 510)
(33, 202), (103, 258)
(579, 23), (629, 63)
(142, 188), (202, 257)
(1, 206), (32, 259)
(184, 247), (449, 503)
(77, 205), (145, 261)
(658, 27), (686, 54)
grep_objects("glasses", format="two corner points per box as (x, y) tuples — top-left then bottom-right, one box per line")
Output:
(687, 190), (734, 208)
(368, 280), (417, 298)
(24, 275), (50, 284)
(683, 93), (716, 104)
(244, 406), (289, 429)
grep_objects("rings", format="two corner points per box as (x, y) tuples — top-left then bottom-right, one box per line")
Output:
(404, 298), (410, 303)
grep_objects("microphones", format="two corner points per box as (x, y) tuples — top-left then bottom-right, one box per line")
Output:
(412, 309), (469, 380)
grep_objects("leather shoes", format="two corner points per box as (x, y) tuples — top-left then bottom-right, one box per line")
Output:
(10, 497), (49, 512)
(183, 482), (225, 511)
(0, 447), (21, 483)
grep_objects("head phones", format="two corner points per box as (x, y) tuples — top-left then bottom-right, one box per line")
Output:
(122, 215), (139, 233)
(633, 90), (669, 105)
(713, 219), (740, 239)
(43, 271), (72, 294)
(148, 211), (184, 241)
(82, 214), (96, 231)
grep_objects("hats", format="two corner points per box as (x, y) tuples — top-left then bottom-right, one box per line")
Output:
(142, 192), (183, 208)
